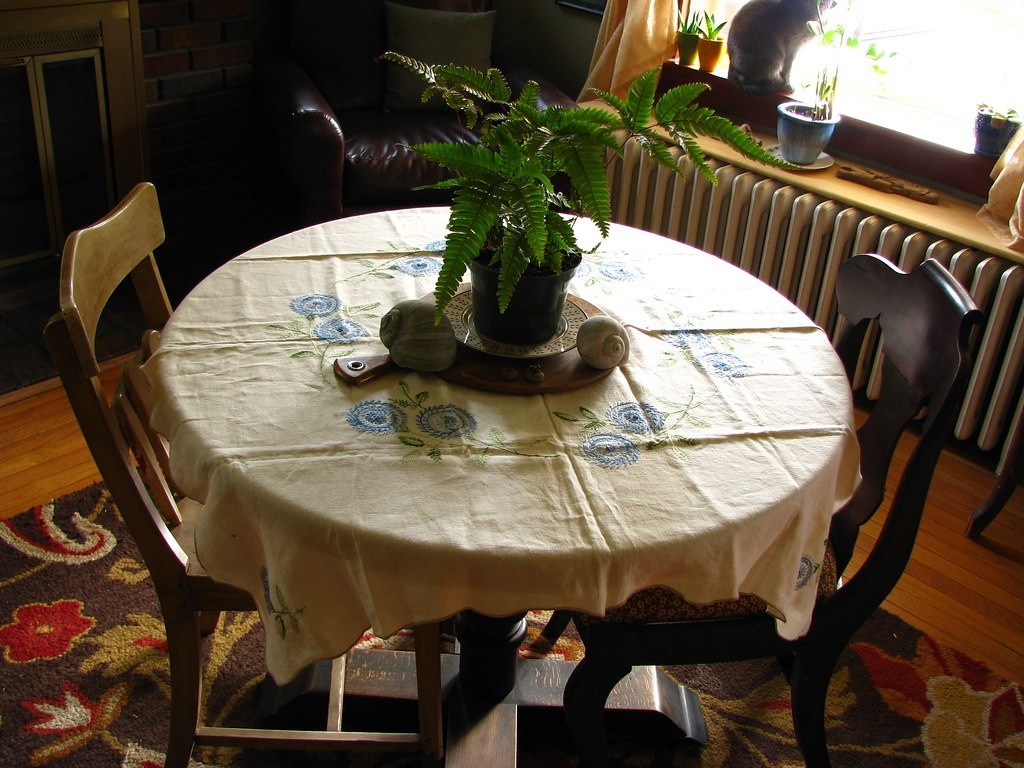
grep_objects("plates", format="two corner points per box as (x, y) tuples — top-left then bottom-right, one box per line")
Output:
(763, 145), (834, 169)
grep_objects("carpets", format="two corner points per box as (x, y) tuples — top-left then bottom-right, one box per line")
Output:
(0, 460), (1023, 767)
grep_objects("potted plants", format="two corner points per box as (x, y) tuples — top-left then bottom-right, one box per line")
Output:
(378, 48), (803, 346)
(677, 10), (702, 64)
(697, 10), (728, 72)
(776, 0), (899, 165)
(974, 102), (1022, 159)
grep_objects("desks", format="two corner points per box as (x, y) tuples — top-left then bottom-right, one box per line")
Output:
(141, 206), (862, 767)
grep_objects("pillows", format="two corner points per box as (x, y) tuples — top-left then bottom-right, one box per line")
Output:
(384, 0), (499, 114)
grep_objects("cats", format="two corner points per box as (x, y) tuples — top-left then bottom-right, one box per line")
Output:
(727, 0), (836, 96)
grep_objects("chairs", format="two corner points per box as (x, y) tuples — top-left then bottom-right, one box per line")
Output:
(40, 182), (445, 768)
(532, 253), (987, 767)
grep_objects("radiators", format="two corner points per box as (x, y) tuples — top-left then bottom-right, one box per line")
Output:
(599, 129), (1024, 452)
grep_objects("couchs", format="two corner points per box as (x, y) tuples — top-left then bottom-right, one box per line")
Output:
(250, 0), (578, 226)
